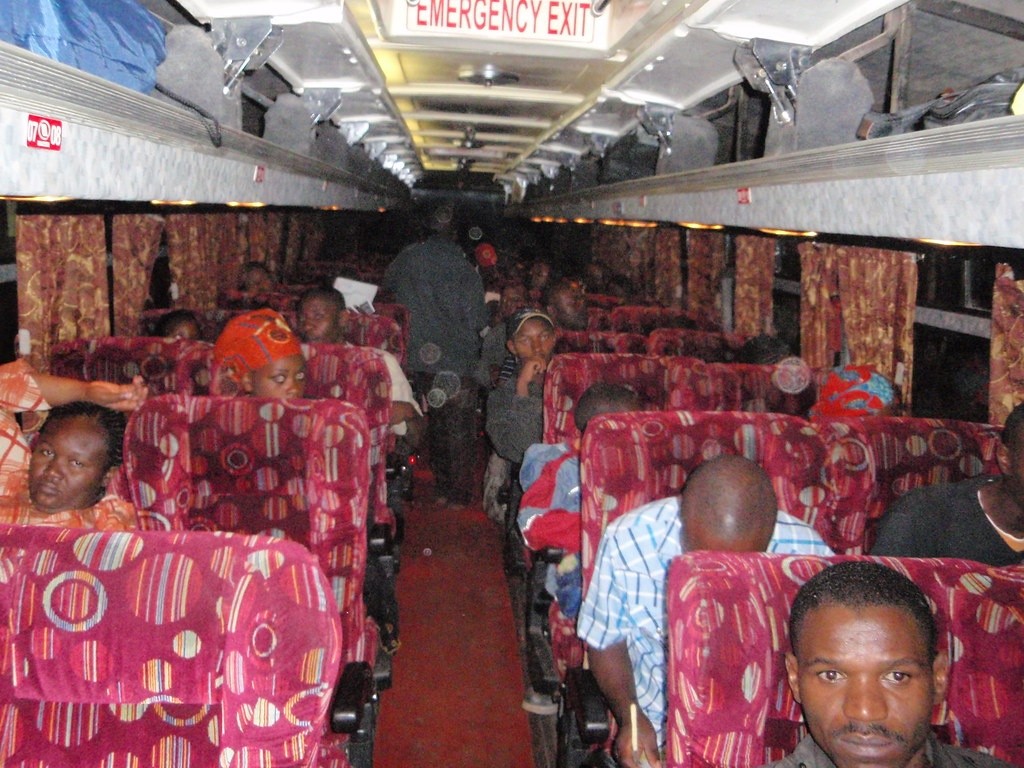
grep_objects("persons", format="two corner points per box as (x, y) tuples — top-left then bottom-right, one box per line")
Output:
(516, 383), (645, 617)
(756, 561), (1018, 767)
(214, 308), (306, 400)
(296, 286), (423, 479)
(480, 238), (636, 331)
(153, 309), (203, 340)
(0, 358), (148, 530)
(484, 309), (557, 463)
(868, 399), (1024, 567)
(236, 261), (283, 310)
(577, 454), (836, 768)
(382, 199), (488, 509)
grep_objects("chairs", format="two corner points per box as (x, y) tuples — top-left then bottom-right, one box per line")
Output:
(1, 258), (1024, 768)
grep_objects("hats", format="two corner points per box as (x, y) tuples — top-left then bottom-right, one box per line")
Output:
(507, 308), (554, 339)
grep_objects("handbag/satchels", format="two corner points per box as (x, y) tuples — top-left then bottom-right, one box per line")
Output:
(0, 0), (223, 148)
(857, 65), (1024, 139)
